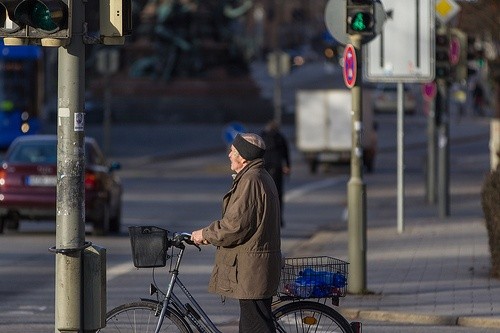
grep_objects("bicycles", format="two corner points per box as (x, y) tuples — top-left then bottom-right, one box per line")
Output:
(95, 226), (363, 333)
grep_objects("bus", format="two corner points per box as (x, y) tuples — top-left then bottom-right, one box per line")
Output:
(1, 37), (58, 147)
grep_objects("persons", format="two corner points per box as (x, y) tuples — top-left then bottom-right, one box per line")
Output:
(258, 121), (291, 229)
(189, 132), (282, 333)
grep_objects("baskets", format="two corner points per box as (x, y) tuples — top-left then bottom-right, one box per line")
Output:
(128, 225), (169, 267)
(277, 256), (350, 299)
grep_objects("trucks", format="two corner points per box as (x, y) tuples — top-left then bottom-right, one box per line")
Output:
(294, 87), (382, 176)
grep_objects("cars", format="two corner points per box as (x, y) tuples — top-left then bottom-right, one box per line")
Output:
(371, 82), (418, 116)
(0, 133), (124, 237)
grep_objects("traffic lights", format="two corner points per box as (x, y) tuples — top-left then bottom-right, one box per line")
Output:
(434, 26), (455, 81)
(448, 28), (478, 83)
(0, 0), (72, 38)
(345, 0), (376, 39)
